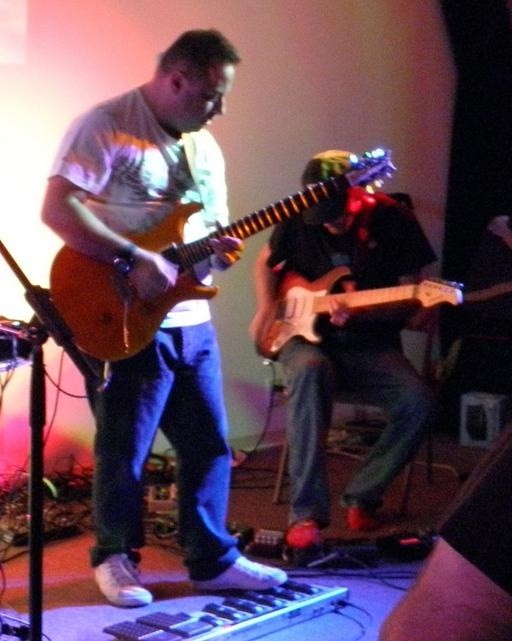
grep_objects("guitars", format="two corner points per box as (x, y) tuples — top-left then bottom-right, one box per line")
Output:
(50, 147), (397, 362)
(256, 267), (464, 362)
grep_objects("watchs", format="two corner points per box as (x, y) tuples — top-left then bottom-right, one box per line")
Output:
(112, 243), (137, 274)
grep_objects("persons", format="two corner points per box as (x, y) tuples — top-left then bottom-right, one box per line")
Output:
(42, 28), (288, 606)
(248, 149), (438, 548)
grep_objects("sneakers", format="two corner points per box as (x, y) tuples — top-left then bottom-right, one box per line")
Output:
(348, 505), (374, 529)
(189, 557), (285, 591)
(286, 523), (320, 548)
(93, 555), (153, 607)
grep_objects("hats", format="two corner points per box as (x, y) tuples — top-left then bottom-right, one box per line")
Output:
(300, 149), (359, 225)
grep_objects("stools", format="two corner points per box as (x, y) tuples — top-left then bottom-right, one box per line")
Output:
(459, 391), (512, 448)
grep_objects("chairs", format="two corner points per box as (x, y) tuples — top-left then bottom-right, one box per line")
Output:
(236, 327), (437, 518)
